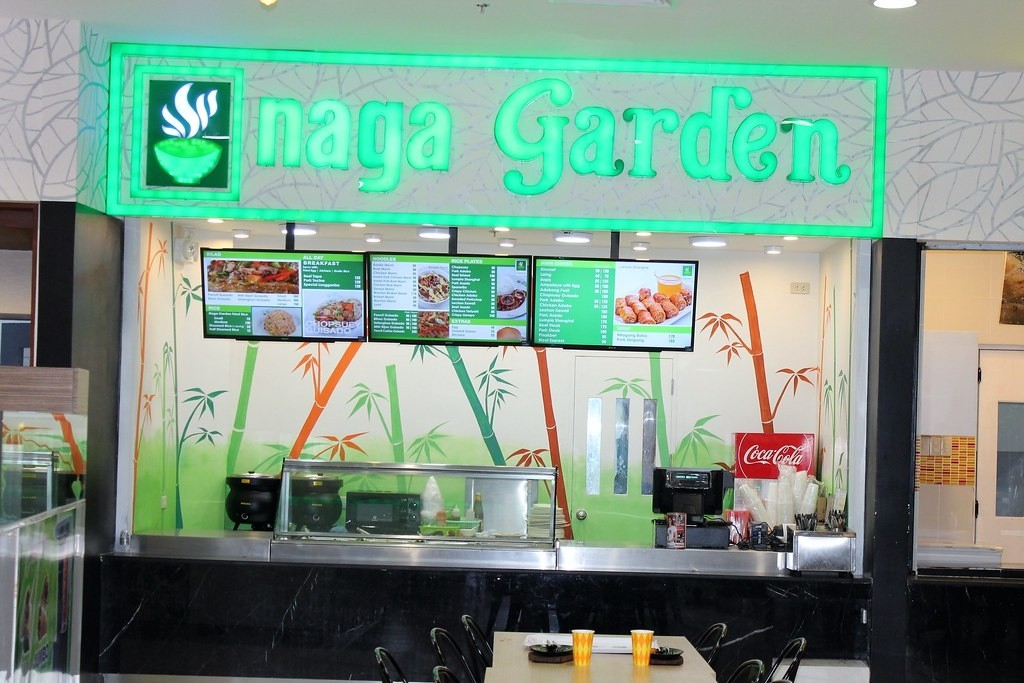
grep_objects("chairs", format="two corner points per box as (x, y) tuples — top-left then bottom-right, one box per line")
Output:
(375, 614), (494, 683)
(688, 622), (806, 683)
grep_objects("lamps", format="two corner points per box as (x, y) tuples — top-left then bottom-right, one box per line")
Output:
(230, 221), (800, 256)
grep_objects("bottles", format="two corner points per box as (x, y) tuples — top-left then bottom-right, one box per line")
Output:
(452, 504), (460, 520)
(474, 493), (484, 532)
(466, 505), (474, 519)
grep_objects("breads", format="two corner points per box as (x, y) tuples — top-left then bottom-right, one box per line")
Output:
(263, 310), (296, 335)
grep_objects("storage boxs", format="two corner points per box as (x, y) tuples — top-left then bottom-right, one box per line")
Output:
(784, 522), (857, 576)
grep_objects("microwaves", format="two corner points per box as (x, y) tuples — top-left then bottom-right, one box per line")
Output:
(345, 491), (423, 535)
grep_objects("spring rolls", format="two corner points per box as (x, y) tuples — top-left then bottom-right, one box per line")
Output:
(615, 288), (692, 324)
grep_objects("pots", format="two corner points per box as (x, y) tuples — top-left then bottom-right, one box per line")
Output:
(225, 471), (282, 530)
(290, 472), (343, 538)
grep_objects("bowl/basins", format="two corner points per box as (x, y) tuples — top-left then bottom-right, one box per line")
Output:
(154, 138), (223, 184)
(461, 529), (475, 537)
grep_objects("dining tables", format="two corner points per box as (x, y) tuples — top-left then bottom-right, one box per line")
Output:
(484, 631), (718, 682)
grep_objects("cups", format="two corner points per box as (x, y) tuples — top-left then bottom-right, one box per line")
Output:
(739, 466), (819, 531)
(572, 629), (595, 666)
(655, 270), (683, 297)
(631, 630), (654, 666)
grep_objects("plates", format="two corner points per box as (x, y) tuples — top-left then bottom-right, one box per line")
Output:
(634, 304), (691, 325)
(525, 504), (571, 539)
(497, 283), (527, 319)
(419, 269), (450, 308)
